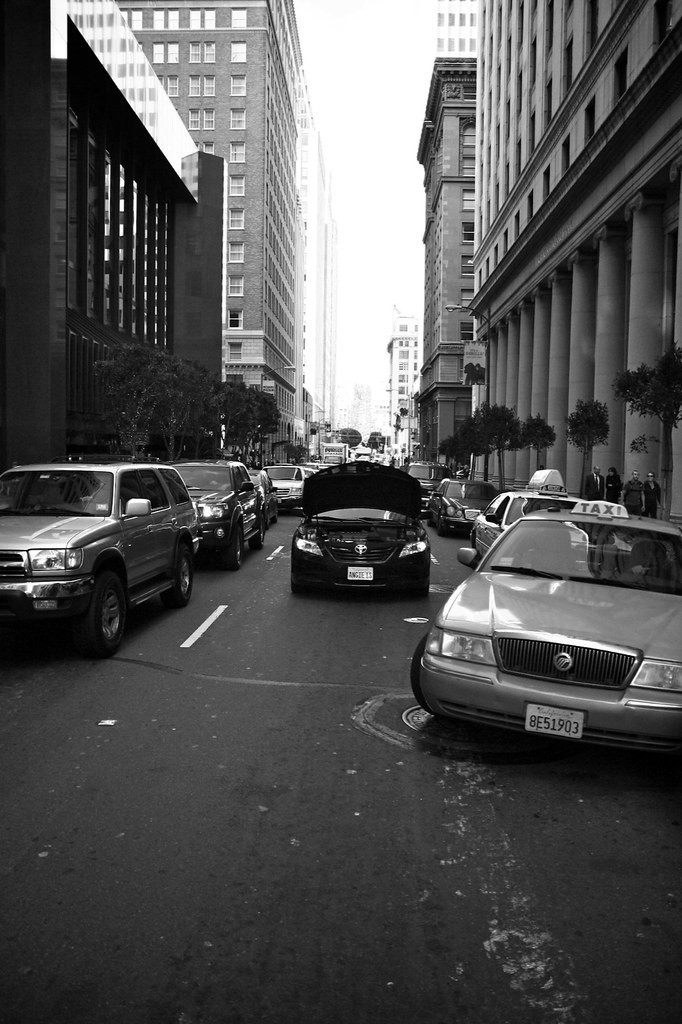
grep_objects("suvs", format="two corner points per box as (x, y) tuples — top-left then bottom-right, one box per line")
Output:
(0, 462), (202, 661)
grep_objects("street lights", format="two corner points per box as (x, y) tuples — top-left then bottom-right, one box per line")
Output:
(385, 388), (412, 462)
(443, 304), (492, 484)
(257, 366), (297, 469)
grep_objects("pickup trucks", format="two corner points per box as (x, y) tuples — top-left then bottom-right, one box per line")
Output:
(168, 459), (266, 570)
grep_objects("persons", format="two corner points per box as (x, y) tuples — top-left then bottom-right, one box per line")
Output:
(585, 465), (604, 501)
(642, 472), (661, 519)
(606, 467), (622, 504)
(621, 470), (645, 516)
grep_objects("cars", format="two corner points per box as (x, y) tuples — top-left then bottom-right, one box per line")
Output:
(464, 469), (594, 566)
(425, 477), (501, 536)
(247, 469), (280, 530)
(409, 499), (682, 759)
(289, 460), (434, 599)
(264, 441), (456, 522)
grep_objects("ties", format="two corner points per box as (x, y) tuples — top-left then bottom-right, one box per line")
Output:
(595, 475), (598, 491)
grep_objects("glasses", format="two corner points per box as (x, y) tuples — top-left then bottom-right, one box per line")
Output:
(647, 476), (654, 477)
